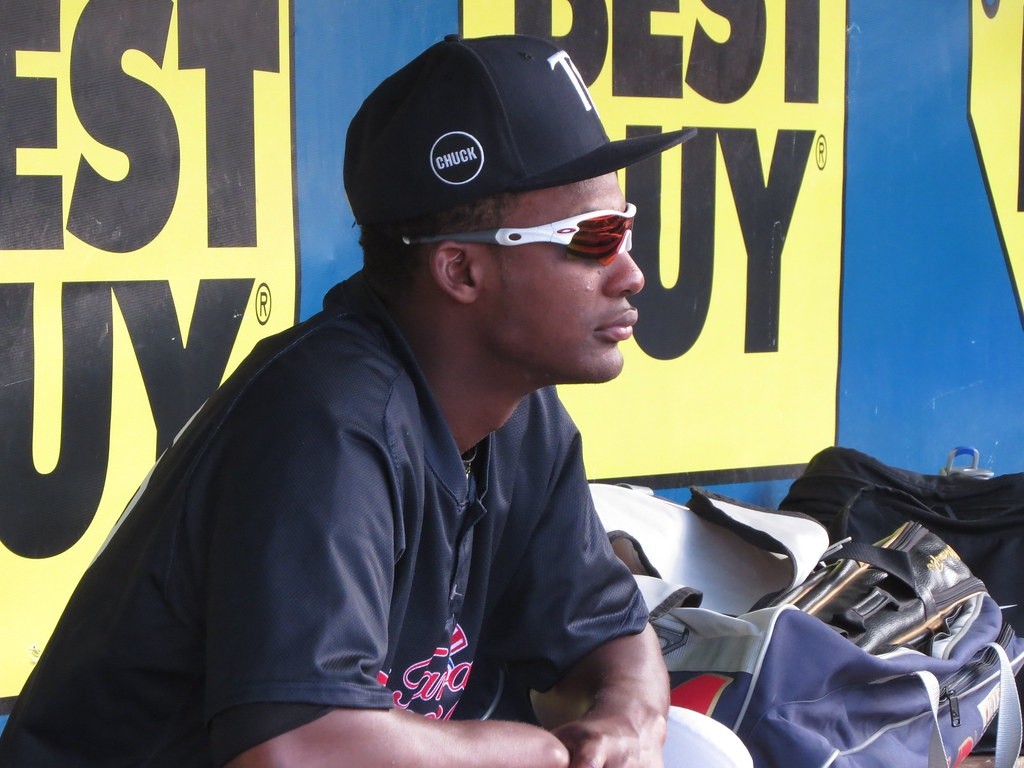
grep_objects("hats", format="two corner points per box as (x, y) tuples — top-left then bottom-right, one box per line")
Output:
(343, 35), (699, 224)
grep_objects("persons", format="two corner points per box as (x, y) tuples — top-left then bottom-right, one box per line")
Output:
(0, 36), (754, 768)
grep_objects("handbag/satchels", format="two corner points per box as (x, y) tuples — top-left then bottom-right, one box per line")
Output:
(533, 446), (1024, 768)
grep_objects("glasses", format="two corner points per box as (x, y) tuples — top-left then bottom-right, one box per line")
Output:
(403, 203), (637, 266)
